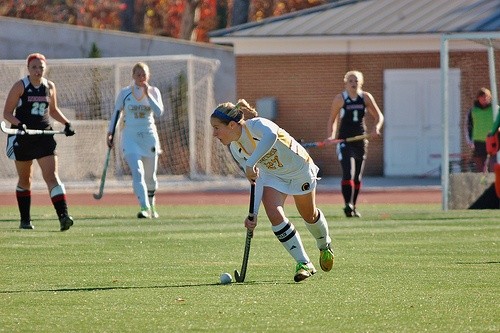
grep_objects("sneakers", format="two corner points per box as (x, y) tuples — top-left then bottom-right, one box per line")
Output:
(294, 260), (317, 282)
(318, 242), (334, 272)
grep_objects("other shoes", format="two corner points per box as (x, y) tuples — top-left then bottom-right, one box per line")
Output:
(353, 207), (361, 217)
(343, 204), (353, 217)
(137, 207), (152, 218)
(59, 213), (73, 231)
(150, 204), (159, 218)
(19, 219), (34, 229)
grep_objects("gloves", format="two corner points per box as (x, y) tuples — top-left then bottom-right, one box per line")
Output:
(64, 122), (75, 137)
(18, 121), (28, 141)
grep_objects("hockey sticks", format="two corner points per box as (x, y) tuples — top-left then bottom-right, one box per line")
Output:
(234, 178), (256, 282)
(0, 121), (77, 134)
(92, 147), (111, 200)
(297, 134), (369, 148)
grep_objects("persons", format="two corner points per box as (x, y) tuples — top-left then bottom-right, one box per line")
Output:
(2, 53), (75, 233)
(209, 99), (334, 282)
(105, 62), (164, 219)
(322, 71), (385, 219)
(466, 87), (500, 174)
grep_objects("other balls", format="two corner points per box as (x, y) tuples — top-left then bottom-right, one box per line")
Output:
(220, 273), (232, 283)
